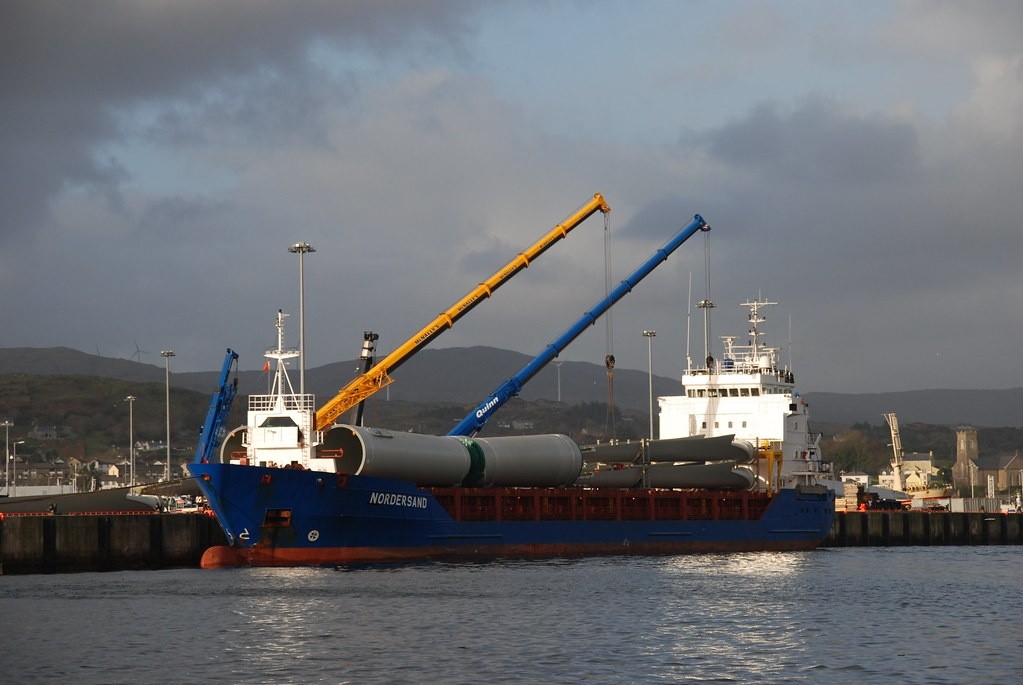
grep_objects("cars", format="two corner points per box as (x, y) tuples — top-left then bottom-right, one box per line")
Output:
(871, 498), (906, 511)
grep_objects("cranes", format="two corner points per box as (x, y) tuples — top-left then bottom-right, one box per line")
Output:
(312, 193), (617, 438)
(446, 215), (713, 437)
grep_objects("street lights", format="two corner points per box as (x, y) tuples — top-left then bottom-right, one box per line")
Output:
(161, 350), (175, 480)
(642, 330), (657, 441)
(13, 440), (26, 497)
(1, 420), (14, 496)
(287, 242), (317, 394)
(123, 395), (137, 496)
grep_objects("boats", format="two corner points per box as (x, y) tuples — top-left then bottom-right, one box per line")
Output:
(187, 291), (837, 569)
